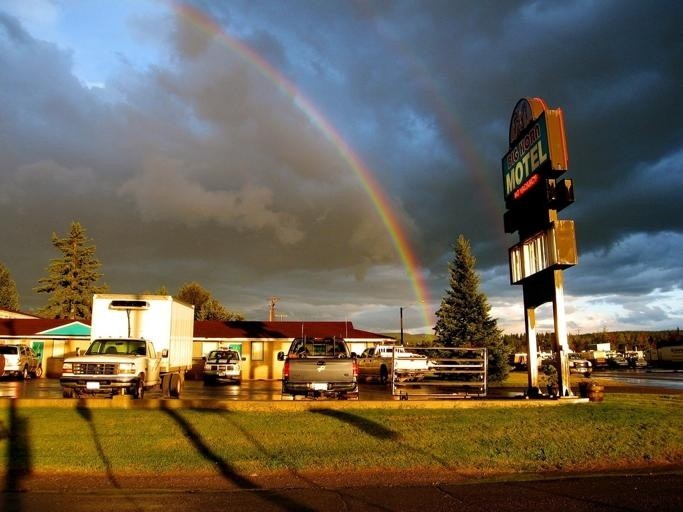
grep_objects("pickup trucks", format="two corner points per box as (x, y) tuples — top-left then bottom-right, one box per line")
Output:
(278, 335), (359, 398)
(357, 346), (428, 385)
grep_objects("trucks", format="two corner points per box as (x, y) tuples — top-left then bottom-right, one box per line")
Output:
(59, 294), (195, 399)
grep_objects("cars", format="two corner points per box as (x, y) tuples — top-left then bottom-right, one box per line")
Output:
(203, 350), (246, 386)
(568, 353), (647, 377)
(0, 345), (43, 379)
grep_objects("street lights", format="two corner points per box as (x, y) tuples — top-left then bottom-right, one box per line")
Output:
(400, 300), (425, 345)
